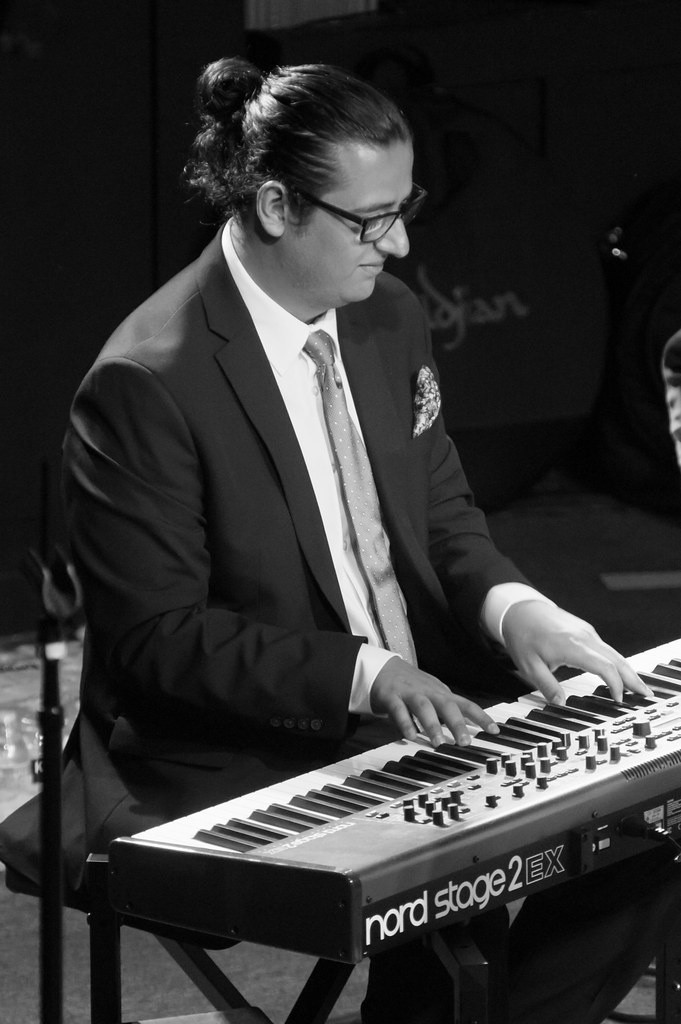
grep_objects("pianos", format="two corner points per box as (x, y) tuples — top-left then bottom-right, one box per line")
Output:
(96, 635), (681, 1024)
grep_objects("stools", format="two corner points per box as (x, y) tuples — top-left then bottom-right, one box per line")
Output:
(0, 772), (273, 1024)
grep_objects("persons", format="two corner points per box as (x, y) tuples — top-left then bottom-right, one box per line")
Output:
(0, 61), (681, 1024)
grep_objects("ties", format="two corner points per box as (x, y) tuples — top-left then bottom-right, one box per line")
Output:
(302, 329), (418, 669)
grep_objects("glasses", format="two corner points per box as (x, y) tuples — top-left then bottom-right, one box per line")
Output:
(291, 182), (428, 243)
